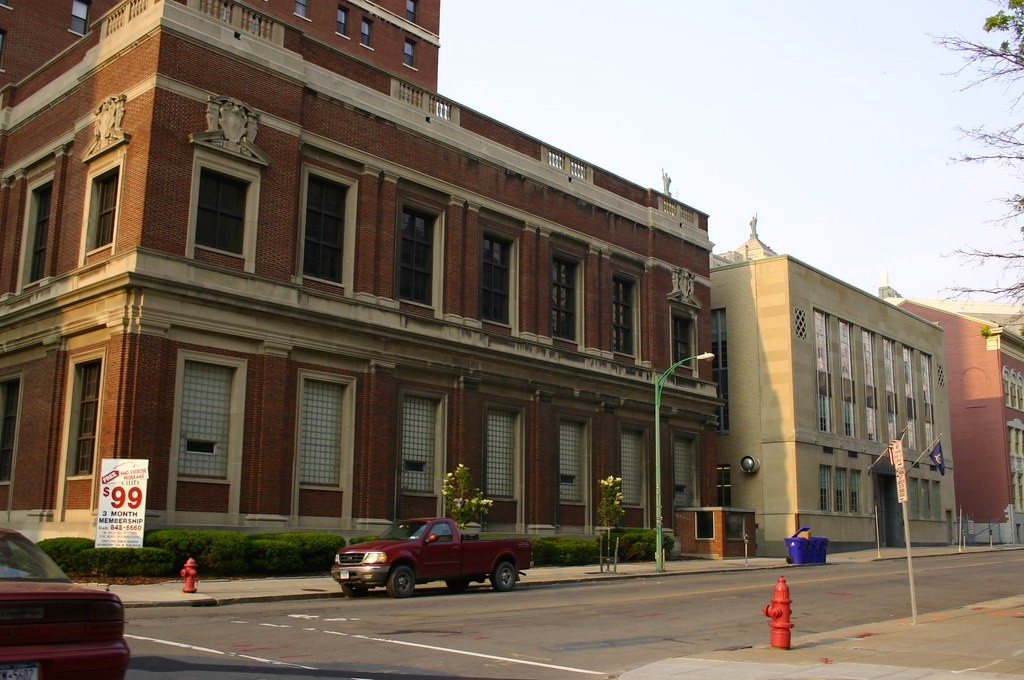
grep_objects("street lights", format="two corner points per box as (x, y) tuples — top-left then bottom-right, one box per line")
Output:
(654, 352), (716, 573)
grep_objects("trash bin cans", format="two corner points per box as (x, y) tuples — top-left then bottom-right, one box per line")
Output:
(819, 537), (829, 563)
(783, 537), (808, 564)
(791, 526), (815, 563)
(811, 536), (823, 562)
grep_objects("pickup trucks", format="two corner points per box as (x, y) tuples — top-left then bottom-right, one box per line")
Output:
(330, 518), (536, 599)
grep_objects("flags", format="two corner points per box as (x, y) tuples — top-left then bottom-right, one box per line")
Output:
(889, 431), (905, 466)
(928, 440), (945, 476)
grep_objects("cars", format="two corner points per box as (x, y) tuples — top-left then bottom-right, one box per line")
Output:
(0, 527), (130, 680)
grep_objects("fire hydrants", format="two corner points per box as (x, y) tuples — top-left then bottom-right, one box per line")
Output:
(762, 575), (795, 649)
(180, 557), (199, 593)
